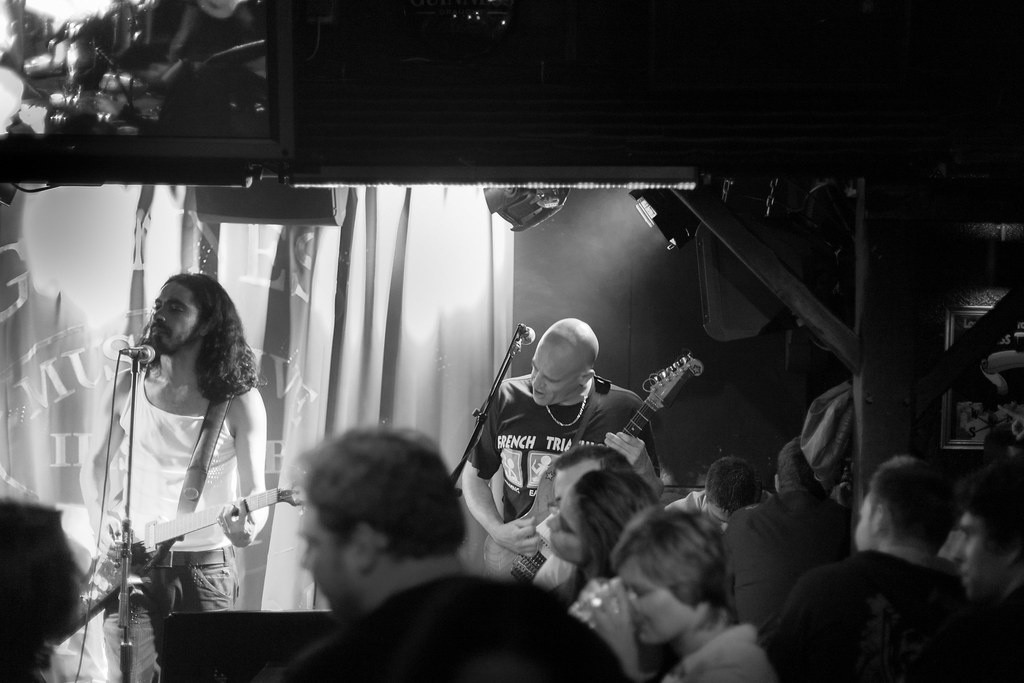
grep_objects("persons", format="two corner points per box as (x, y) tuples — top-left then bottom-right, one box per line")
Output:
(1, 498), (86, 683)
(717, 434), (852, 652)
(532, 445), (635, 592)
(948, 429), (1018, 528)
(907, 484), (1024, 683)
(816, 455), (852, 510)
(546, 469), (680, 683)
(279, 423), (628, 683)
(665, 455), (776, 537)
(80, 274), (270, 683)
(463, 316), (665, 585)
(579, 502), (779, 683)
(757, 455), (989, 683)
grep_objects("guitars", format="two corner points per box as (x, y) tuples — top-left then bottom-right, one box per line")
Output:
(47, 483), (300, 647)
(482, 353), (703, 582)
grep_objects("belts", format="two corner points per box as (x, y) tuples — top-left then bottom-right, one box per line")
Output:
(146, 548), (238, 569)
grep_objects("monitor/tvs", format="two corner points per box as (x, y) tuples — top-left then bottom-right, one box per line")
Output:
(1, 0), (295, 161)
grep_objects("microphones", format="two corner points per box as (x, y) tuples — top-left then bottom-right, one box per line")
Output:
(120, 345), (155, 365)
(517, 323), (536, 346)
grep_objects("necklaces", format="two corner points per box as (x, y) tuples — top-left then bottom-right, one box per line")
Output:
(545, 393), (586, 427)
